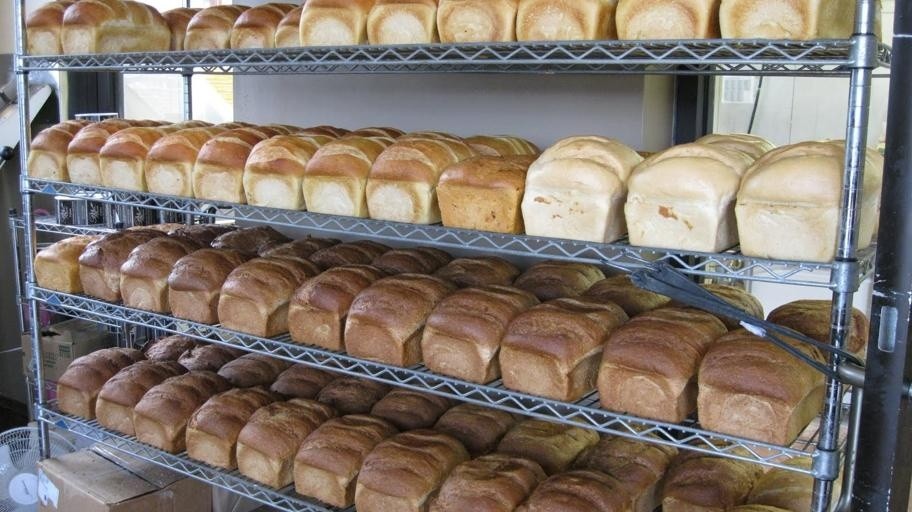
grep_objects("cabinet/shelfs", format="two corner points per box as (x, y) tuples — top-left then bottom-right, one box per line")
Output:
(13, 1), (895, 512)
(7, 208), (58, 422)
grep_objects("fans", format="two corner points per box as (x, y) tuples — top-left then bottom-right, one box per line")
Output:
(0, 426), (78, 512)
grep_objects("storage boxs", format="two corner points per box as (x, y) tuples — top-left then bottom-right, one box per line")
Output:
(38, 437), (212, 512)
(20, 318), (116, 384)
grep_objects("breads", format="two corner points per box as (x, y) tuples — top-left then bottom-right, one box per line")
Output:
(421, 282), (537, 387)
(177, 342), (247, 371)
(515, 1), (616, 40)
(695, 323), (831, 456)
(32, 234), (99, 294)
(718, 1), (886, 41)
(29, 118), (288, 203)
(292, 413), (398, 509)
(161, 6), (199, 50)
(659, 437), (766, 511)
(513, 261), (605, 300)
(230, 3), (298, 48)
(92, 358), (187, 435)
(434, 403), (523, 455)
(209, 224), (291, 255)
(56, 345), (146, 420)
(366, 0), (437, 44)
(436, 134), (659, 246)
(438, 254), (521, 289)
(622, 127), (886, 263)
(132, 371), (231, 456)
(78, 228), (166, 303)
(571, 424), (678, 511)
(588, 272), (670, 314)
(166, 248), (255, 325)
(521, 468), (629, 512)
(318, 375), (389, 414)
(298, 0), (372, 45)
(345, 272), (460, 368)
(61, 1), (171, 53)
(694, 283), (765, 320)
(286, 264), (388, 352)
(130, 222), (187, 234)
(371, 246), (453, 273)
(498, 414), (603, 474)
(216, 350), (292, 388)
(140, 336), (190, 359)
(436, 0), (516, 42)
(354, 429), (471, 512)
(235, 396), (339, 490)
(271, 358), (342, 401)
(184, 4), (252, 50)
(595, 307), (725, 421)
(120, 236), (207, 313)
(220, 254), (318, 338)
(267, 234), (340, 258)
(24, 1), (74, 55)
(371, 380), (457, 428)
(615, 0), (717, 38)
(272, 8), (303, 46)
(184, 386), (282, 469)
(766, 298), (869, 375)
(311, 239), (391, 266)
(240, 125), (539, 224)
(168, 222), (240, 246)
(498, 296), (626, 402)
(751, 456), (838, 512)
(429, 453), (545, 512)
